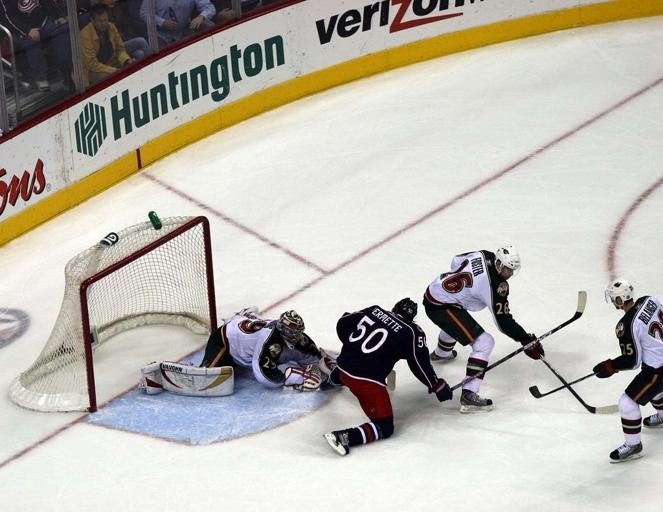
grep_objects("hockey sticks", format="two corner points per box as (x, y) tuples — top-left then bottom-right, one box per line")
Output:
(529, 370), (600, 398)
(539, 354), (618, 414)
(449, 291), (587, 392)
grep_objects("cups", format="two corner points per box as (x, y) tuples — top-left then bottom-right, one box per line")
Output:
(98, 231), (118, 249)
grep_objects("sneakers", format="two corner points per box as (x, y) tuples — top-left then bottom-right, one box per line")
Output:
(430, 350), (457, 361)
(36, 78), (50, 90)
(643, 414), (663, 426)
(333, 428), (354, 454)
(460, 389), (492, 406)
(320, 379), (334, 390)
(610, 441), (642, 459)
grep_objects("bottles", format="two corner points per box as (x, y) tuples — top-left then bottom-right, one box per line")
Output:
(147, 211), (162, 229)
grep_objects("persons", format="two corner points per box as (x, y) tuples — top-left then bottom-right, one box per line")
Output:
(422, 243), (545, 407)
(327, 298), (453, 455)
(0, 0), (233, 93)
(199, 307), (322, 390)
(593, 279), (663, 461)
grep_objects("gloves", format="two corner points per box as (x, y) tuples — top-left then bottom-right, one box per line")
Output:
(592, 359), (618, 378)
(521, 334), (544, 359)
(434, 381), (452, 402)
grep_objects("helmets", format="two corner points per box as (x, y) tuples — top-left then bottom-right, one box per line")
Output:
(494, 245), (520, 279)
(605, 279), (634, 310)
(276, 310), (305, 345)
(392, 297), (417, 323)
